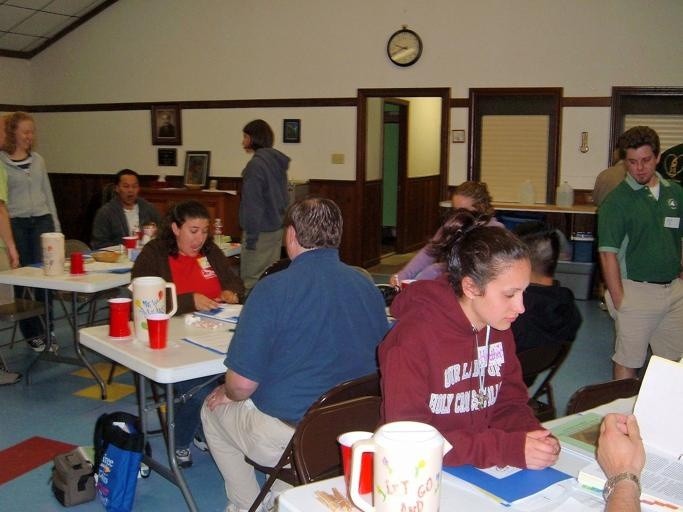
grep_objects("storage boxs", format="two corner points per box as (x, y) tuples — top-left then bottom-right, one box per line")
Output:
(569, 230), (597, 259)
(498, 217), (547, 238)
(552, 261), (597, 302)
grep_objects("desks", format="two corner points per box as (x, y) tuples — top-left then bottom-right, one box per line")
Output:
(436, 198), (599, 217)
(0, 235), (243, 402)
(277, 392), (683, 512)
(79, 295), (401, 511)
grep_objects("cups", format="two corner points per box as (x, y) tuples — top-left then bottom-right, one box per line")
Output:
(123, 223), (157, 260)
(147, 314), (169, 348)
(40, 232), (65, 275)
(71, 253), (83, 275)
(106, 297), (132, 337)
(336, 429), (375, 494)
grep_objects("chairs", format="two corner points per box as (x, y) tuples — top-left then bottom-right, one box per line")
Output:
(294, 395), (385, 482)
(58, 239), (121, 336)
(0, 249), (57, 349)
(241, 370), (378, 512)
(514, 338), (580, 420)
(564, 375), (639, 413)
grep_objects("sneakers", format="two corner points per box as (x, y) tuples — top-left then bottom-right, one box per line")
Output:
(26, 330), (62, 354)
(175, 446), (192, 467)
(194, 435), (212, 450)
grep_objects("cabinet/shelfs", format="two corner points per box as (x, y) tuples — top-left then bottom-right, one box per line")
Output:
(104, 183), (240, 241)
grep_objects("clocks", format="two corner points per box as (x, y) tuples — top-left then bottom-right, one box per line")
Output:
(386, 24), (423, 65)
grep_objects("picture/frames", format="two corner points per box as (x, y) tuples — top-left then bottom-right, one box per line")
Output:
(281, 117), (302, 145)
(451, 129), (466, 143)
(183, 149), (210, 189)
(150, 103), (182, 147)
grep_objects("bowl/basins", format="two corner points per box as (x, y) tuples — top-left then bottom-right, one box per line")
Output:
(91, 252), (121, 263)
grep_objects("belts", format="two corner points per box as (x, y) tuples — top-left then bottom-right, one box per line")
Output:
(630, 277), (675, 286)
(273, 417), (304, 429)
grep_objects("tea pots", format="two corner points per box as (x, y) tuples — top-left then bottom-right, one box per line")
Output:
(127, 276), (176, 334)
(348, 420), (454, 512)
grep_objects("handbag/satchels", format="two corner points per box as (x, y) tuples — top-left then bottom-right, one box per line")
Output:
(50, 411), (144, 512)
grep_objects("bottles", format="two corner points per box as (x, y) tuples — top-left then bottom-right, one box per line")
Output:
(213, 218), (224, 250)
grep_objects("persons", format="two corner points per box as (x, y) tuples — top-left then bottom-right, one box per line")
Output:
(5, 112), (61, 352)
(0, 117), (22, 386)
